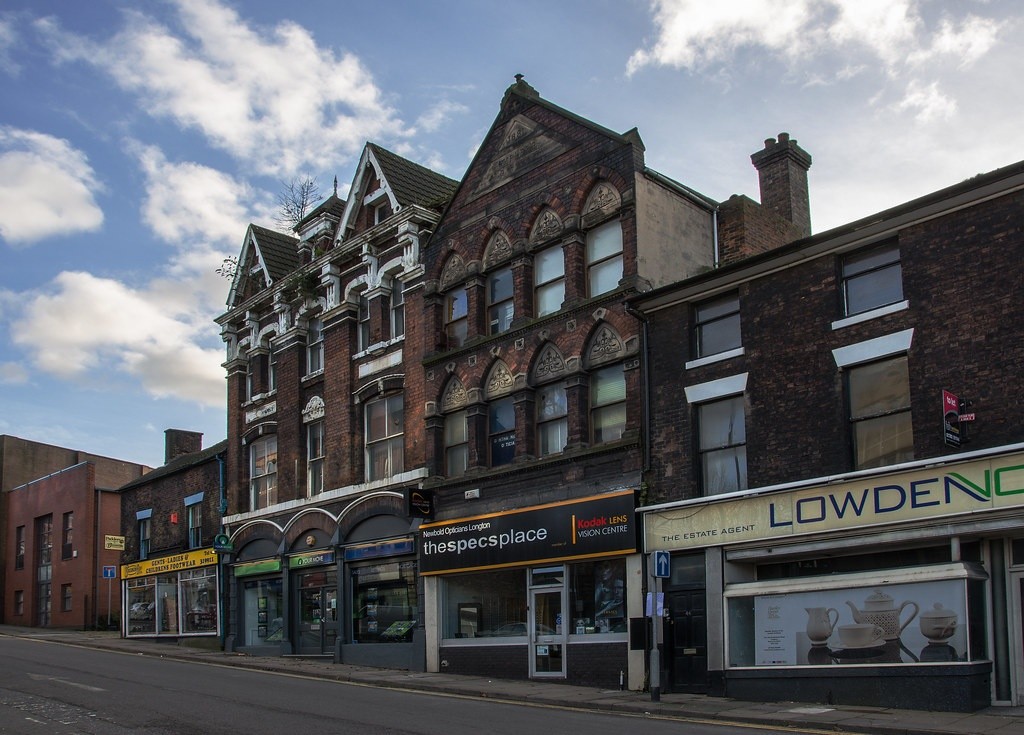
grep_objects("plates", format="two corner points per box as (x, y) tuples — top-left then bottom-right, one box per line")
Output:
(829, 641), (887, 650)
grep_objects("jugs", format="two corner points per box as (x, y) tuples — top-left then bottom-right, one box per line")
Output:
(804, 606), (839, 645)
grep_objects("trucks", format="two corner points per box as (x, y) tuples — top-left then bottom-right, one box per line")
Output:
(358, 606), (413, 624)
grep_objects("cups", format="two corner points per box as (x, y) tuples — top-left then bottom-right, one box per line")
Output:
(921, 603), (958, 643)
(836, 622), (884, 647)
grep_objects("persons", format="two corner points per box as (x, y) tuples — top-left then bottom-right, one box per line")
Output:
(595, 563), (623, 617)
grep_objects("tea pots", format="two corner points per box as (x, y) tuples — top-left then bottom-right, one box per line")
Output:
(844, 590), (919, 642)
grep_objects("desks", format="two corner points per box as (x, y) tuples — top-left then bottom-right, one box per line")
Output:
(184, 613), (211, 632)
(130, 618), (154, 634)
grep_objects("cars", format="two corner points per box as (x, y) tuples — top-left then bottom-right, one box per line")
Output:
(493, 622), (554, 637)
(130, 602), (154, 622)
(185, 592), (216, 628)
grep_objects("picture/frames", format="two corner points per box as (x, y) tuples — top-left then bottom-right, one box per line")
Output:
(458, 602), (482, 639)
(257, 596), (267, 610)
(257, 625), (267, 637)
(257, 611), (267, 623)
